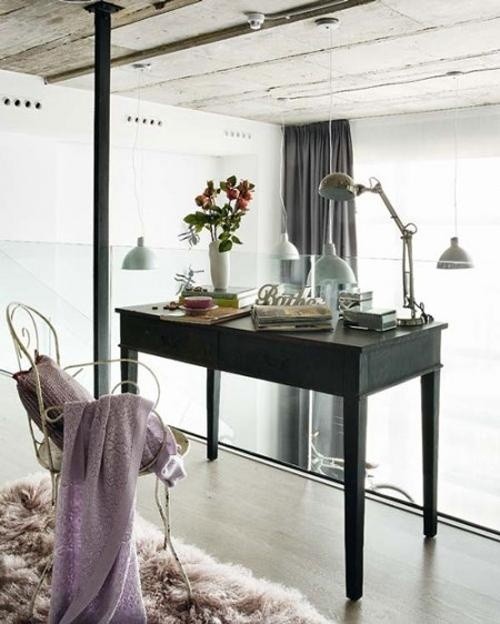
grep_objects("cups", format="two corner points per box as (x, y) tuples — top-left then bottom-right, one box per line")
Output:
(320, 279), (339, 319)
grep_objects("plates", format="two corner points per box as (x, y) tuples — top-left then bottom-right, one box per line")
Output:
(179, 304), (219, 314)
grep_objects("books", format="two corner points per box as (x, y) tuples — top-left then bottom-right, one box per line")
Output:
(160, 306), (250, 325)
(250, 305), (335, 333)
(179, 296), (259, 309)
(181, 287), (260, 299)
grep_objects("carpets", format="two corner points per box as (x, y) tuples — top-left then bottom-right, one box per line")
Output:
(0, 470), (336, 622)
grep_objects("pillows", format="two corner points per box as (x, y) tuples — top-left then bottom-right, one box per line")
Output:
(8, 347), (95, 455)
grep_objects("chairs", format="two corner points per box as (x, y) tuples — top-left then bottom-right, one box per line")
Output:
(0, 296), (203, 623)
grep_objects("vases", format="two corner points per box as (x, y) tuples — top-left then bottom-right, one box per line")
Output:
(208, 239), (234, 293)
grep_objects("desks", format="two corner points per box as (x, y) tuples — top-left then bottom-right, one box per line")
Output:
(111, 297), (449, 605)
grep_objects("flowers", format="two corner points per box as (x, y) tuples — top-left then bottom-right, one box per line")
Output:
(184, 168), (257, 259)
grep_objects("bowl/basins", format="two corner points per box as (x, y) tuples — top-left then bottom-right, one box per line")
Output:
(184, 296), (212, 308)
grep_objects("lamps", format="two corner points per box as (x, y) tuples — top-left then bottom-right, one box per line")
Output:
(273, 93), (301, 264)
(316, 170), (429, 326)
(305, 15), (361, 291)
(435, 65), (476, 274)
(116, 58), (166, 276)
